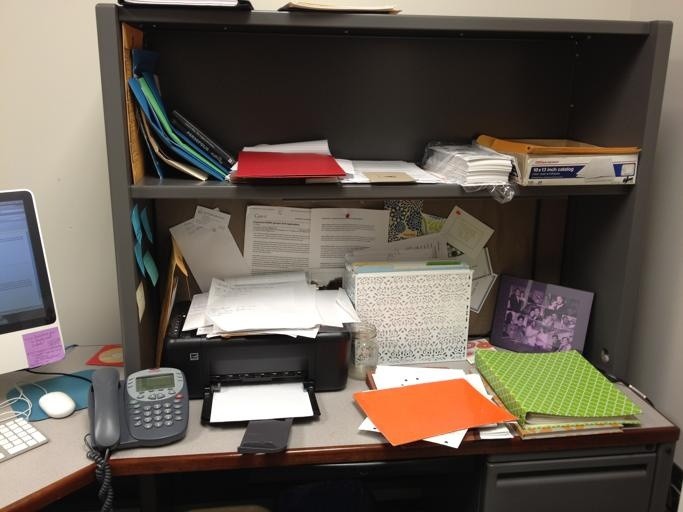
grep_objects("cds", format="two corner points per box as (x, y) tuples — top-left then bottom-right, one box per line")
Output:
(98, 347), (123, 364)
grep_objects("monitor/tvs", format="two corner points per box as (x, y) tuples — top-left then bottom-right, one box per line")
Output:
(0, 188), (65, 376)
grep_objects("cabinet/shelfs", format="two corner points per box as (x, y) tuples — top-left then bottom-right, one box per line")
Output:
(96, 3), (674, 383)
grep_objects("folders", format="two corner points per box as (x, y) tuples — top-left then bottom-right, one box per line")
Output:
(474, 348), (644, 441)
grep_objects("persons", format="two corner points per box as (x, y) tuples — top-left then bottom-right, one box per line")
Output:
(503, 285), (576, 353)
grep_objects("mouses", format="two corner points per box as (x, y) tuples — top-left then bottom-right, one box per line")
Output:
(38, 391), (76, 418)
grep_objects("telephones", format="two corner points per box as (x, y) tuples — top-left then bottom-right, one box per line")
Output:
(88, 367), (189, 454)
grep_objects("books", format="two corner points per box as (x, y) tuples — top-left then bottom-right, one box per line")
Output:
(362, 351), (642, 439)
(115, 0), (254, 10)
(278, 1), (401, 15)
(121, 24), (236, 185)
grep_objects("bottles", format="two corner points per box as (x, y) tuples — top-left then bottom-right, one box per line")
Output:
(348, 323), (378, 381)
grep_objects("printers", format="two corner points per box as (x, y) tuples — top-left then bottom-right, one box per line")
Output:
(161, 299), (351, 424)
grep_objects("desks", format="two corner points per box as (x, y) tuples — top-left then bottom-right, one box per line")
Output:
(0, 346), (683, 511)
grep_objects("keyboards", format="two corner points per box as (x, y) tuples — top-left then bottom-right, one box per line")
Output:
(0, 417), (48, 462)
